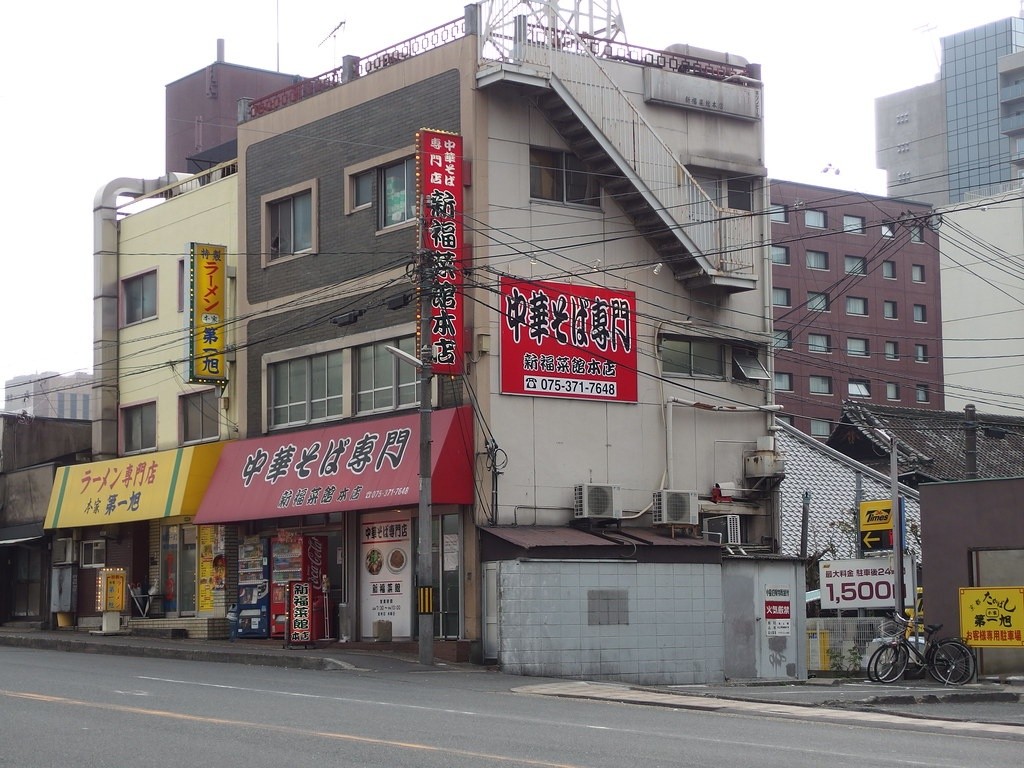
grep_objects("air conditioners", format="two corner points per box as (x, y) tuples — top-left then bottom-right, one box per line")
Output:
(573, 483), (623, 519)
(652, 490), (698, 525)
(703, 515), (740, 544)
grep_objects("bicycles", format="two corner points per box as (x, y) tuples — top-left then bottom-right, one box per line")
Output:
(868, 608), (977, 686)
